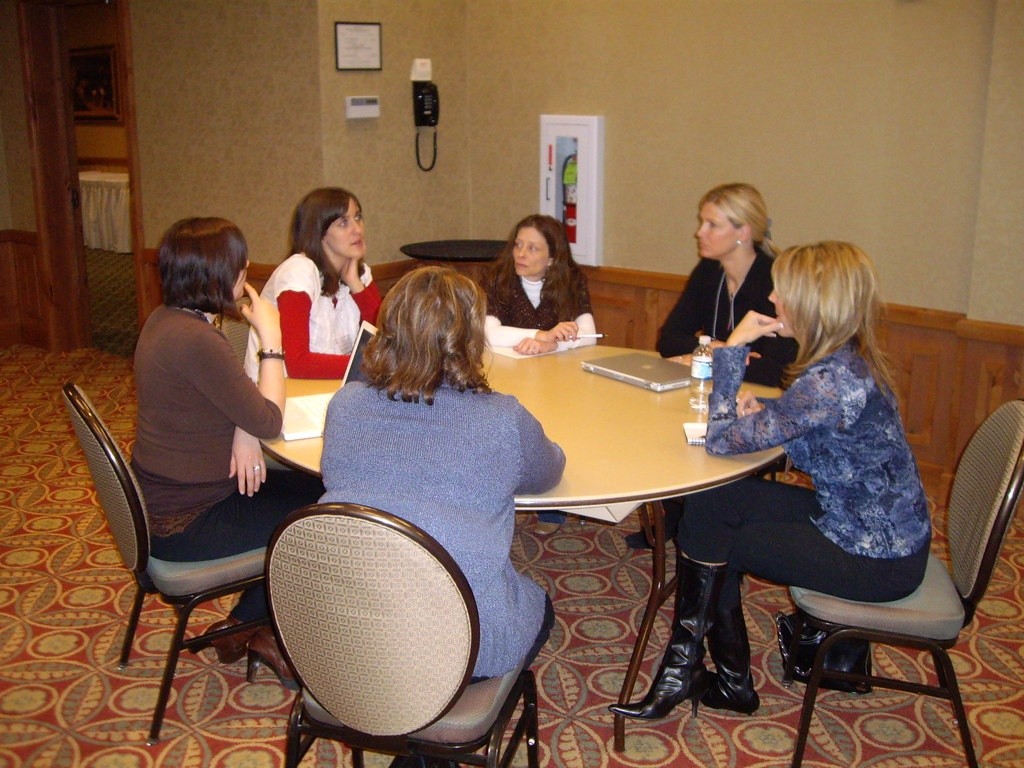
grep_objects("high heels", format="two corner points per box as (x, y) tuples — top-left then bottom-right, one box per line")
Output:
(188, 617), (264, 664)
(245, 629), (300, 689)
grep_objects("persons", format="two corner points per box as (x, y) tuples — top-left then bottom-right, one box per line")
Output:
(245, 187), (382, 382)
(317, 265), (566, 768)
(623, 184), (801, 550)
(607, 240), (932, 726)
(131, 218), (327, 690)
(479, 215), (597, 535)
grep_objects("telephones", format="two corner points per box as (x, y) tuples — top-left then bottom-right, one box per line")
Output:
(412, 82), (439, 126)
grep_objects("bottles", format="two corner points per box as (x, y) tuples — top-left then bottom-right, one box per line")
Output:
(691, 335), (714, 415)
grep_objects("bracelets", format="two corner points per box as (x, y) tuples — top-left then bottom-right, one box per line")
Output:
(258, 349), (284, 361)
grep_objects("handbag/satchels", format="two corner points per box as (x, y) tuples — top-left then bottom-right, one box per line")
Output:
(773, 608), (872, 694)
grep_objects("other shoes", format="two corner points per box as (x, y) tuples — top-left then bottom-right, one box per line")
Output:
(624, 518), (678, 548)
(534, 520), (559, 537)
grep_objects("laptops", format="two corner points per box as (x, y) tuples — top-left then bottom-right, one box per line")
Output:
(281, 321), (380, 442)
(581, 354), (694, 391)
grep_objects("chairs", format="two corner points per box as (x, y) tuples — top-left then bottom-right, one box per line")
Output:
(61, 383), (270, 741)
(263, 503), (540, 768)
(790, 398), (1024, 767)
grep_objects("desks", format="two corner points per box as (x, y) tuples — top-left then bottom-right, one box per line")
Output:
(259, 344), (787, 754)
(399, 239), (510, 262)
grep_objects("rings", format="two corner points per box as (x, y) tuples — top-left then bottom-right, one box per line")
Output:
(253, 465), (260, 471)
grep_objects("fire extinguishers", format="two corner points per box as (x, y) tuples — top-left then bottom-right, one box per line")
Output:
(562, 151), (577, 243)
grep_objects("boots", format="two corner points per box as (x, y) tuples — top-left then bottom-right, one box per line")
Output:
(699, 561), (760, 717)
(608, 551), (729, 720)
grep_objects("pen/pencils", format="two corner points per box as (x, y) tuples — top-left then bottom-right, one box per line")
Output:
(570, 334), (609, 338)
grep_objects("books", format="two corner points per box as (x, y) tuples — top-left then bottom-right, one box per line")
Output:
(683, 422), (707, 446)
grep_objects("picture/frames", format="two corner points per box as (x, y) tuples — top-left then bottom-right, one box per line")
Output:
(334, 21), (383, 72)
(70, 44), (122, 127)
